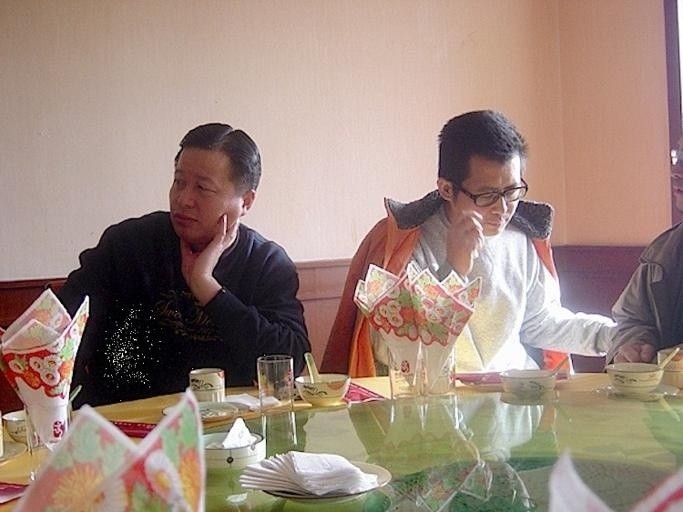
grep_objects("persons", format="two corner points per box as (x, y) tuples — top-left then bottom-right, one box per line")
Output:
(636, 392), (683, 478)
(200, 409), (311, 512)
(604, 131), (683, 367)
(317, 107), (615, 378)
(54, 122), (311, 411)
(340, 390), (554, 511)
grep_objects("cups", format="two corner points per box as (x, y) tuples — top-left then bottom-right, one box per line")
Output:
(261, 411), (297, 448)
(391, 395), (459, 429)
(386, 341), (456, 401)
(658, 348), (683, 390)
(190, 367), (226, 402)
(257, 355), (295, 414)
(24, 402), (73, 484)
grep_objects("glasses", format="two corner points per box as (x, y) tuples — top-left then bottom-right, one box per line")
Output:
(451, 177), (529, 208)
(670, 147), (683, 166)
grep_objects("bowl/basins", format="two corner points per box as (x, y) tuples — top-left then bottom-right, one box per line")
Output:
(296, 374), (351, 408)
(1, 410), (27, 444)
(605, 362), (664, 397)
(498, 369), (558, 402)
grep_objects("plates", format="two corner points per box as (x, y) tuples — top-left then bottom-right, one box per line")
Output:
(1, 441), (27, 466)
(162, 401), (250, 423)
(263, 459), (393, 503)
